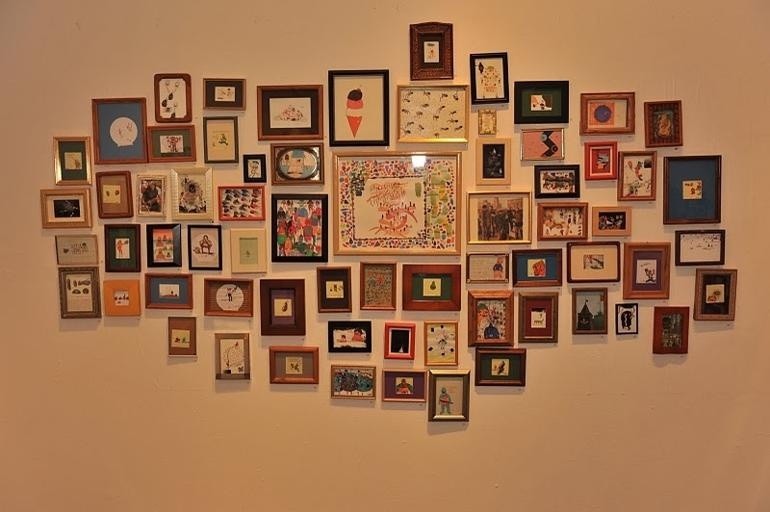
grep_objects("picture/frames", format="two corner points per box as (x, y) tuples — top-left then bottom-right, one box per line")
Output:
(410, 21), (456, 79)
(332, 152), (463, 254)
(427, 369), (470, 422)
(91, 73), (247, 164)
(691, 268), (737, 323)
(474, 138), (512, 184)
(615, 150), (657, 201)
(641, 99), (681, 148)
(401, 264), (461, 311)
(583, 141), (619, 182)
(422, 320), (459, 368)
(468, 52), (508, 108)
(255, 84), (325, 139)
(592, 206), (634, 238)
(582, 92), (636, 134)
(466, 291), (514, 346)
(512, 79), (571, 125)
(326, 320), (373, 355)
(314, 267), (351, 315)
(378, 368), (427, 403)
(40, 135), (268, 319)
(477, 108), (499, 136)
(538, 202), (589, 241)
(465, 190), (532, 245)
(534, 163), (579, 198)
(271, 143), (325, 187)
(672, 230), (727, 265)
(328, 363), (376, 401)
(517, 291), (558, 344)
(512, 248), (564, 287)
(518, 127), (565, 163)
(464, 252), (510, 282)
(326, 71), (389, 147)
(395, 85), (469, 143)
(476, 349), (528, 389)
(662, 158), (722, 226)
(383, 321), (418, 361)
(270, 196), (326, 262)
(565, 242), (690, 355)
(166, 275), (318, 386)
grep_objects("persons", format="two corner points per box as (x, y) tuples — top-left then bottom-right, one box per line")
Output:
(438, 387), (451, 414)
(352, 330), (363, 341)
(663, 317), (680, 348)
(200, 235), (212, 254)
(226, 287), (233, 303)
(396, 379), (413, 394)
(492, 257), (505, 279)
(144, 181), (161, 211)
(645, 268), (656, 283)
(478, 202), (523, 239)
(73, 159), (82, 170)
(118, 240), (124, 257)
